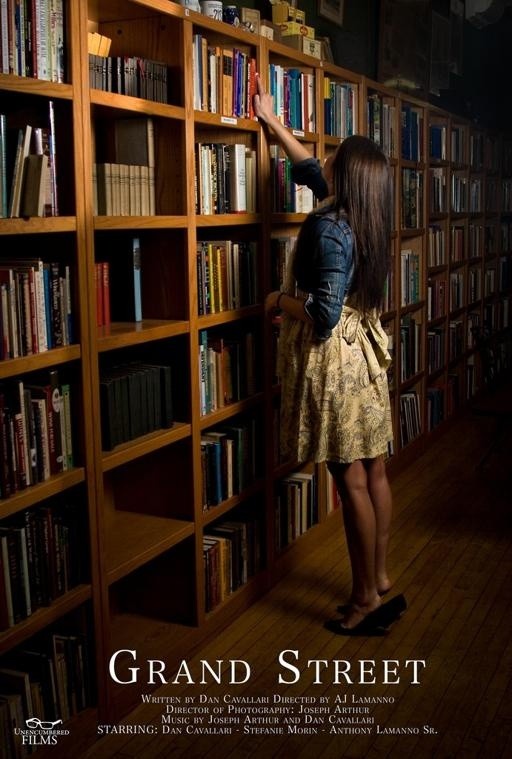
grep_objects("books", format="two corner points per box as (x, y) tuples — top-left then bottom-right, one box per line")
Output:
(0, 0), (512, 741)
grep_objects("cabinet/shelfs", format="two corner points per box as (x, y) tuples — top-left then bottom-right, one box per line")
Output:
(0, 0), (106, 758)
(84, 0), (322, 720)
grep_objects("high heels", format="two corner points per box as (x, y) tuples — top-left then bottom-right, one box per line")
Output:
(324, 594), (408, 637)
